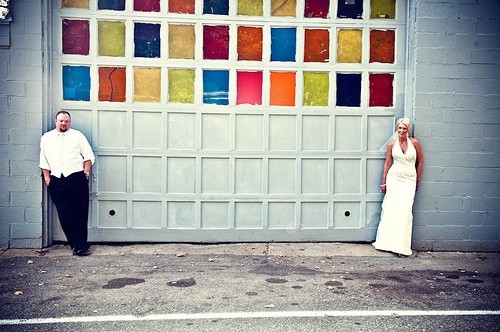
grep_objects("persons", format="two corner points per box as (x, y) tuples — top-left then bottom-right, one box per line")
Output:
(370, 117), (423, 256)
(39, 110), (95, 258)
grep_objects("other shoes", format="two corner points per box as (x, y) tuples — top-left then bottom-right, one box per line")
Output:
(77, 244), (91, 254)
(73, 248), (79, 255)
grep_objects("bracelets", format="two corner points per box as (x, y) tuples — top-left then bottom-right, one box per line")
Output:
(85, 172), (91, 177)
(380, 184), (386, 188)
